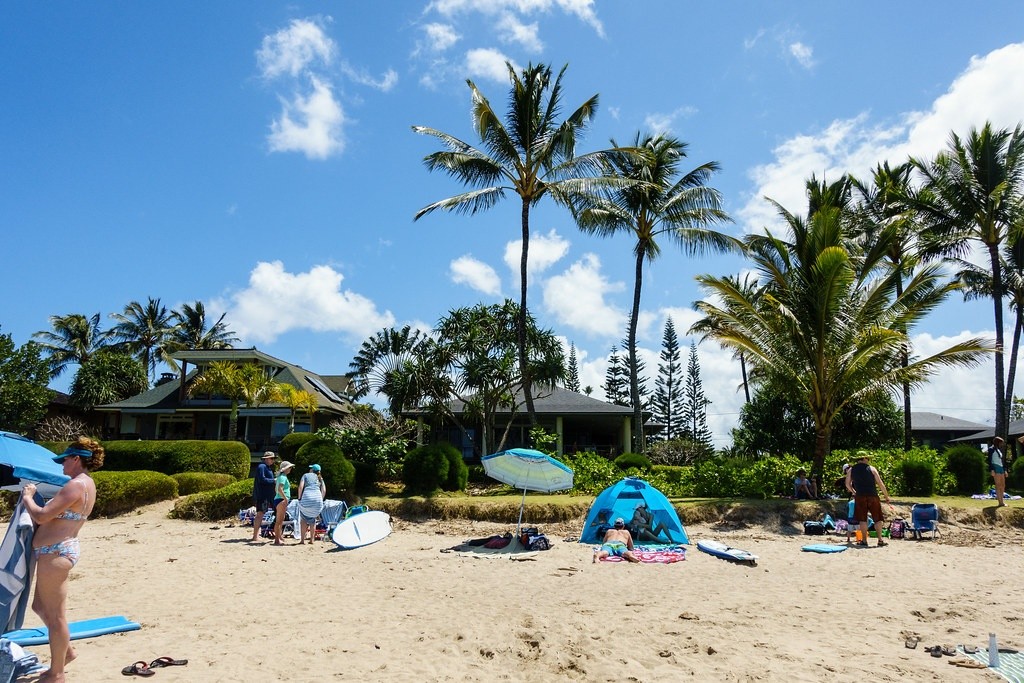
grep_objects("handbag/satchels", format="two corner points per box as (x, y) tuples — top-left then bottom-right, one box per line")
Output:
(485, 527), (550, 551)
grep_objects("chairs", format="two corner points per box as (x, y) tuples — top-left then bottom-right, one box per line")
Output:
(238, 497), (370, 541)
(905, 504), (941, 542)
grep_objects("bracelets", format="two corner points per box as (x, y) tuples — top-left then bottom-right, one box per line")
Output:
(298, 498), (301, 501)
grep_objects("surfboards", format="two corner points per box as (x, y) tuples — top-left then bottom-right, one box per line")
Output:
(802, 543), (848, 553)
(331, 511), (395, 550)
(696, 539), (760, 562)
(1, 615), (141, 646)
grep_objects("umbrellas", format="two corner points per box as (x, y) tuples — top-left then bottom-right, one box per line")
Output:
(1, 431), (72, 499)
(481, 447), (573, 539)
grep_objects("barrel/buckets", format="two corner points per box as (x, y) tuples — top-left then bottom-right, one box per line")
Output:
(855, 530), (869, 543)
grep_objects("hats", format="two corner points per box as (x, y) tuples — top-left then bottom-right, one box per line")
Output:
(843, 464), (849, 474)
(852, 451), (873, 459)
(261, 451), (278, 458)
(279, 461), (295, 472)
(796, 467), (807, 473)
(636, 505), (646, 510)
(51, 448), (92, 464)
(309, 464), (321, 471)
(614, 518), (624, 526)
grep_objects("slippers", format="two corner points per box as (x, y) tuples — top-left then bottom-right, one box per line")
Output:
(148, 657), (188, 669)
(122, 661), (154, 677)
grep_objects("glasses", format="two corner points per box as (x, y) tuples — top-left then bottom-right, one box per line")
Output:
(801, 474), (806, 476)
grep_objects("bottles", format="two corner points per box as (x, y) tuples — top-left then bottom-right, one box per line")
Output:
(989, 633), (999, 666)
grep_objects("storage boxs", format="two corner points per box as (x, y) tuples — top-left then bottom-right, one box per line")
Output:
(804, 520), (826, 535)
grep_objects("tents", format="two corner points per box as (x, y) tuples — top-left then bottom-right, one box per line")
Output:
(578, 477), (690, 545)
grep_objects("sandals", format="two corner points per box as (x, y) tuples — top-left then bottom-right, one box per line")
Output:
(857, 541), (867, 545)
(878, 540), (890, 546)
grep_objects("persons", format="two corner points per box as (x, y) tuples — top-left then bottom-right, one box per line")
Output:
(272, 460), (295, 545)
(794, 470), (820, 501)
(843, 464), (856, 495)
(22, 435), (105, 683)
(297, 463), (326, 545)
(252, 451), (279, 541)
(846, 450), (890, 546)
(629, 503), (682, 544)
(988, 437), (1008, 508)
(593, 518), (641, 563)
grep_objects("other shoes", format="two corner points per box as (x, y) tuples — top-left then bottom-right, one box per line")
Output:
(905, 637), (988, 668)
(814, 496), (821, 500)
(1000, 503), (1007, 507)
(809, 496), (816, 500)
